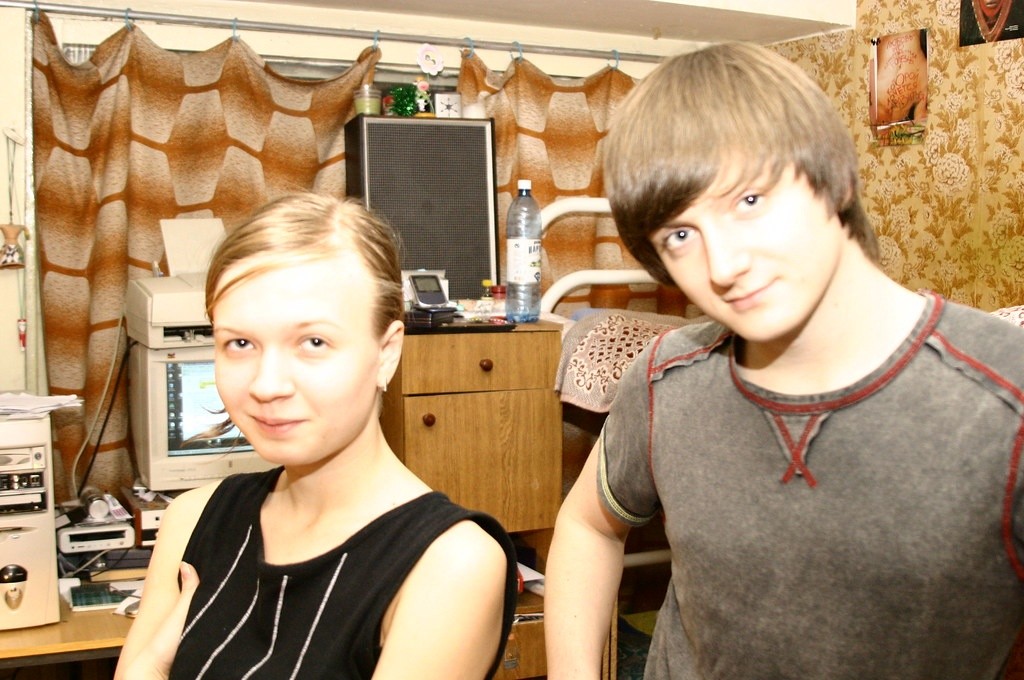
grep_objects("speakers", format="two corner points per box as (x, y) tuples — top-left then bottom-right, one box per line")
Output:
(344, 113), (499, 313)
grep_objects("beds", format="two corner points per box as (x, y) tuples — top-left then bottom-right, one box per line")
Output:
(540, 196), (1024, 680)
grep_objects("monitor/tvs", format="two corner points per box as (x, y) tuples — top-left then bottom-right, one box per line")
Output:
(125, 341), (286, 492)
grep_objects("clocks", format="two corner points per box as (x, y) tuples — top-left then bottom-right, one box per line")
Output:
(433, 92), (461, 118)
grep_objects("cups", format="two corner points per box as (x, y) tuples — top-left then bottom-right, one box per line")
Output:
(352, 87), (382, 116)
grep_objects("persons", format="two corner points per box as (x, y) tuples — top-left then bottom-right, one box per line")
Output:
(544, 42), (1024, 680)
(113, 192), (516, 680)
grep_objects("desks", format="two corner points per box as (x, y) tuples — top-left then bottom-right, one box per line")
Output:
(0, 568), (148, 680)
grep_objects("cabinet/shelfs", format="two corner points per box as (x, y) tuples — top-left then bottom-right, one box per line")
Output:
(379, 322), (564, 680)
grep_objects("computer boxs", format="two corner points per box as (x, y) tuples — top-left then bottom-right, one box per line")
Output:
(0, 413), (60, 630)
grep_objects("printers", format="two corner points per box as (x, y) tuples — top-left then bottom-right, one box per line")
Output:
(124, 274), (216, 350)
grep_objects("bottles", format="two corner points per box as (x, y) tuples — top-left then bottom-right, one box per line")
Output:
(491, 286), (505, 315)
(78, 485), (109, 519)
(505, 180), (542, 324)
(480, 280), (494, 303)
